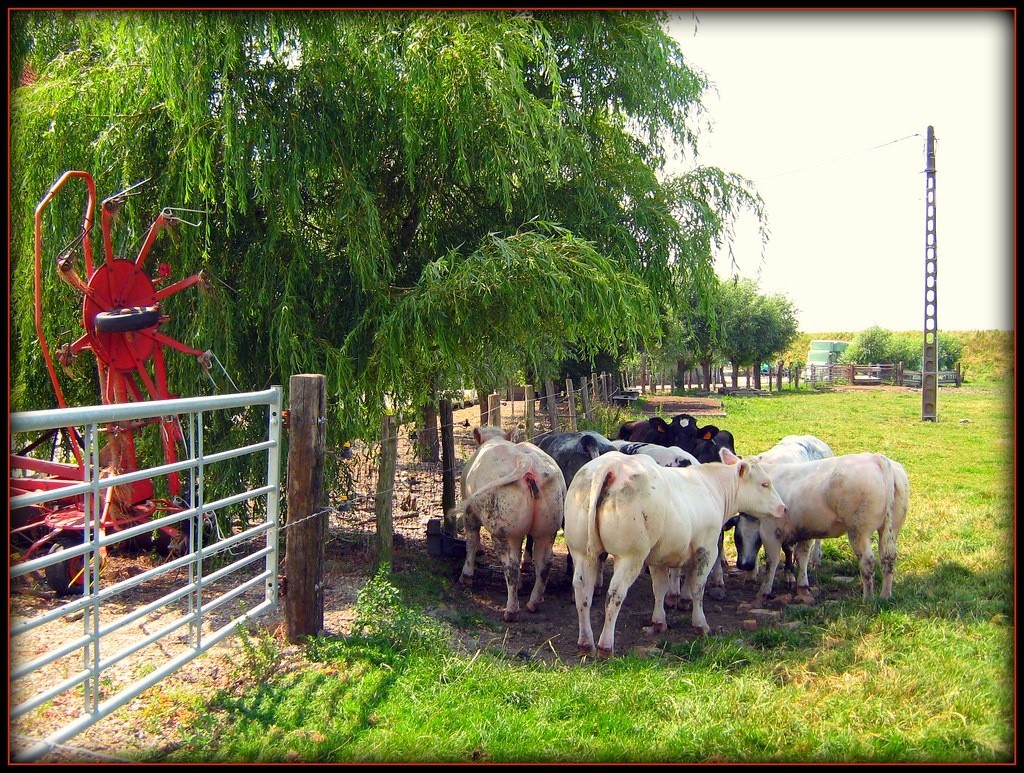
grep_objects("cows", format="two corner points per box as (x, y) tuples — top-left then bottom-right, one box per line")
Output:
(445, 413), (910, 660)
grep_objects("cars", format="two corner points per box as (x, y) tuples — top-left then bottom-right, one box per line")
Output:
(340, 373), (499, 435)
